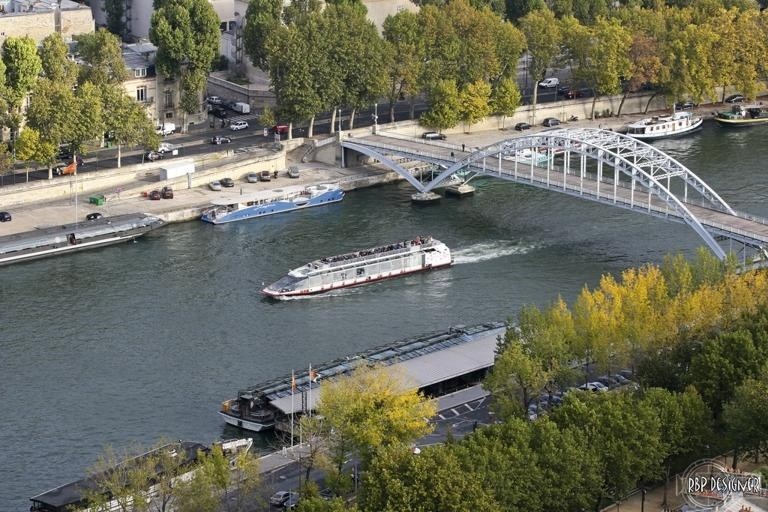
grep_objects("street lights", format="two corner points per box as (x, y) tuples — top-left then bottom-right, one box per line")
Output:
(614, 496), (622, 512)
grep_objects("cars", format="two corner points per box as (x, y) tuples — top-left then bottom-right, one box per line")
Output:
(280, 492), (303, 509)
(150, 190), (160, 200)
(268, 490), (296, 505)
(207, 94), (306, 146)
(54, 145), (85, 178)
(538, 76), (585, 100)
(247, 172), (257, 183)
(146, 122), (176, 163)
(0, 212), (11, 222)
(85, 212), (104, 221)
(514, 122), (531, 131)
(725, 95), (744, 104)
(286, 165), (300, 178)
(222, 178), (235, 187)
(521, 364), (638, 426)
(260, 171), (270, 181)
(674, 100), (694, 111)
(209, 180), (222, 191)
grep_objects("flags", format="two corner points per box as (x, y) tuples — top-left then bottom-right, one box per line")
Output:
(310, 367), (321, 382)
(292, 377), (297, 392)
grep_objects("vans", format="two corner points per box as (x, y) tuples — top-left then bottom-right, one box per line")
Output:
(421, 133), (446, 141)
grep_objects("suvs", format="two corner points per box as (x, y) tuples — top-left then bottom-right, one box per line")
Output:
(162, 187), (173, 199)
(543, 118), (560, 127)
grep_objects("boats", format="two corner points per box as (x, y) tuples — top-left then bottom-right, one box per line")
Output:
(717, 103), (768, 131)
(262, 235), (454, 299)
(28, 435), (256, 512)
(431, 130), (622, 185)
(202, 182), (345, 225)
(626, 111), (704, 142)
(0, 209), (167, 268)
